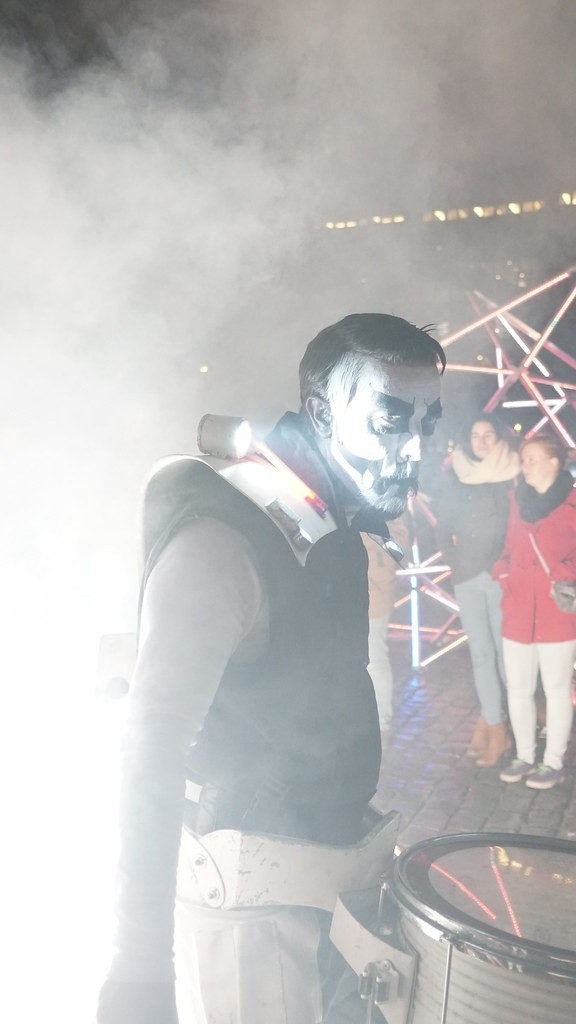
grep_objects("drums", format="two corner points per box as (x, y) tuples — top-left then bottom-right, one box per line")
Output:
(369, 829), (576, 1024)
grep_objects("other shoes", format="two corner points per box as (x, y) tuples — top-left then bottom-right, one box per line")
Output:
(381, 728), (397, 748)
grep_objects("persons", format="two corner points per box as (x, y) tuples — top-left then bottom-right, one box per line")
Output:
(93, 313), (448, 1024)
(359, 412), (576, 788)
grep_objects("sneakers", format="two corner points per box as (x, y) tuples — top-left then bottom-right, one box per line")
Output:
(499, 759), (537, 782)
(525, 764), (565, 789)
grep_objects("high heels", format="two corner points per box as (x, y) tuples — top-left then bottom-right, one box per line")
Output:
(476, 723), (512, 768)
(466, 716), (490, 755)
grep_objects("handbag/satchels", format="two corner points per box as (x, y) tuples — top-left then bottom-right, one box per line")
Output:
(550, 581), (576, 613)
